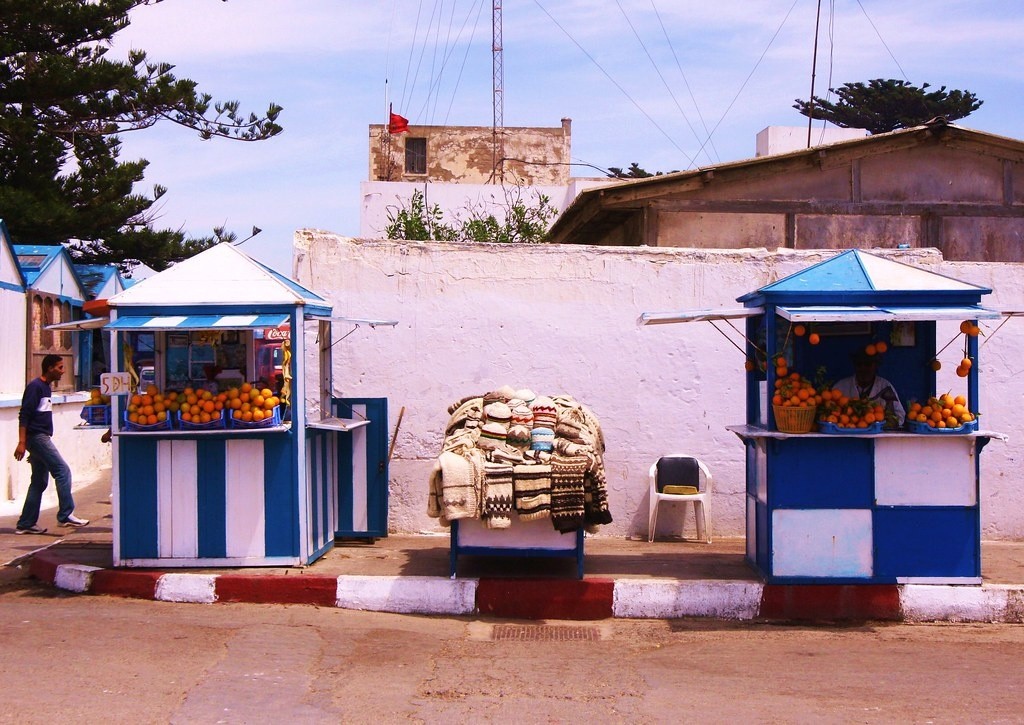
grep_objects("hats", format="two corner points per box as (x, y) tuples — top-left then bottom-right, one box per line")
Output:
(483, 402), (511, 427)
(478, 422), (507, 441)
(508, 426), (531, 452)
(511, 406), (534, 427)
(514, 389), (536, 404)
(533, 398), (557, 429)
(848, 347), (871, 359)
(507, 399), (526, 409)
(556, 410), (582, 437)
(531, 427), (555, 450)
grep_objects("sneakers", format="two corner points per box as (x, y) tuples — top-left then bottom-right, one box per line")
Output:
(15, 524), (48, 534)
(56, 515), (90, 528)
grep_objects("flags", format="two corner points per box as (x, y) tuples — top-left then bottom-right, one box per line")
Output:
(389, 114), (410, 133)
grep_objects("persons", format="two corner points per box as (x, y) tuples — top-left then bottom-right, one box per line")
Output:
(13, 354), (90, 535)
(832, 346), (906, 425)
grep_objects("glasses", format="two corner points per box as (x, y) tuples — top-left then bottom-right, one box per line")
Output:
(854, 361), (872, 367)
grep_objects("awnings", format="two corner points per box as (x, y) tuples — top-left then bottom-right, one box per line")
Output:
(104, 314), (288, 329)
(42, 317), (109, 331)
(307, 315), (400, 351)
(776, 304), (1002, 354)
(936, 305), (1024, 356)
(637, 306), (766, 357)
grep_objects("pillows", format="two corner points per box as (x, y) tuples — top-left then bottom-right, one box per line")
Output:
(657, 457), (699, 492)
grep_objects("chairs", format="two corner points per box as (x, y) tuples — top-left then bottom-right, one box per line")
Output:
(648, 454), (713, 543)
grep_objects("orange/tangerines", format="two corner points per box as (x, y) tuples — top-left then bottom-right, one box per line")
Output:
(128, 383), (280, 425)
(84, 388), (110, 405)
(743, 321), (979, 429)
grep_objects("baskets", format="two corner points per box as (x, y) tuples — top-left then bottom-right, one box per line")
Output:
(229, 405), (282, 429)
(772, 404), (817, 433)
(178, 408), (226, 429)
(124, 408), (173, 431)
(907, 420), (977, 434)
(819, 420), (886, 435)
(80, 404), (110, 426)
